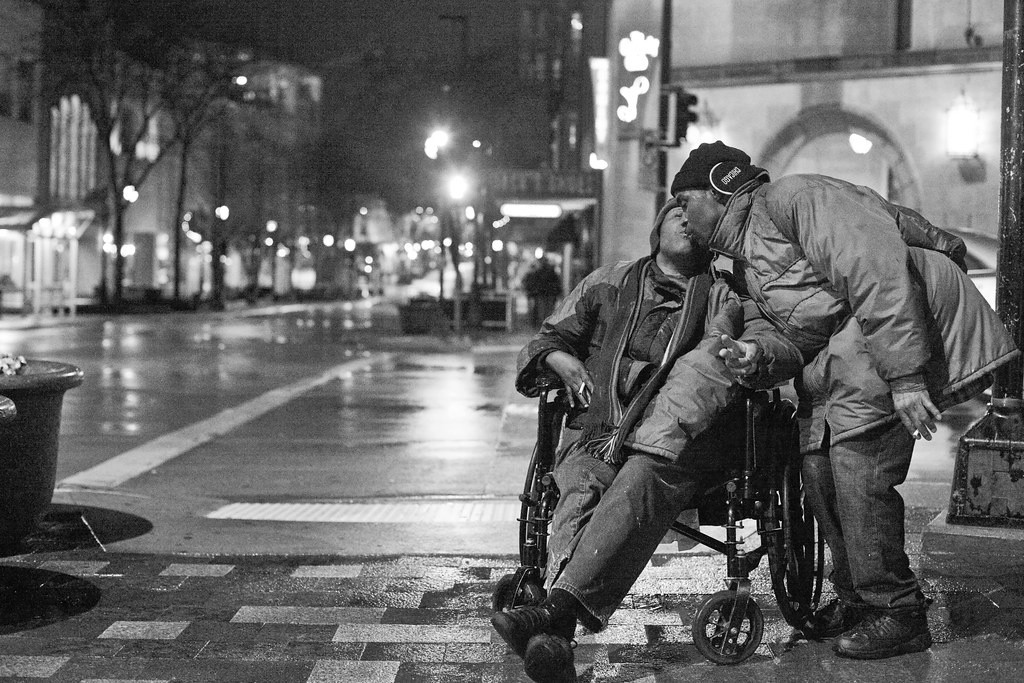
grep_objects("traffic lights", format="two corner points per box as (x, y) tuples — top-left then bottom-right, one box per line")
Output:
(660, 84), (700, 148)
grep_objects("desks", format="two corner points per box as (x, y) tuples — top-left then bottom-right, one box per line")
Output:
(449, 293), (516, 333)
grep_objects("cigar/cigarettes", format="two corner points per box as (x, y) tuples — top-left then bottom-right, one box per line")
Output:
(579, 382), (586, 396)
(912, 430), (920, 438)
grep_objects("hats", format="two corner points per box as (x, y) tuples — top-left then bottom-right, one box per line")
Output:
(671, 140), (751, 198)
(648, 198), (680, 258)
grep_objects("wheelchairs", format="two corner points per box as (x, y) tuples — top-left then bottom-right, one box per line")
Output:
(491, 381), (826, 667)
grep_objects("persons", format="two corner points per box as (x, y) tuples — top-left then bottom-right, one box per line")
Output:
(669, 141), (1022, 658)
(491, 192), (819, 683)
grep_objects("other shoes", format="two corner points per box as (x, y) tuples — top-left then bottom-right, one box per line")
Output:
(491, 607), (577, 659)
(524, 633), (577, 683)
(814, 598), (869, 636)
(832, 609), (933, 660)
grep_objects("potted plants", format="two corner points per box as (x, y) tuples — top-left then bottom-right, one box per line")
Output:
(0, 356), (82, 546)
(399, 294), (438, 334)
